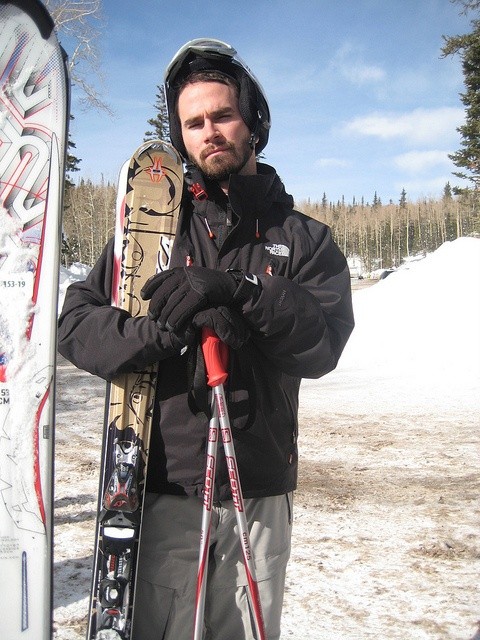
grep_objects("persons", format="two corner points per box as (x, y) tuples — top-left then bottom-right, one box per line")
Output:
(57, 37), (356, 640)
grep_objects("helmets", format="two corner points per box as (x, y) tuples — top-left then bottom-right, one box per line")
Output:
(165, 39), (270, 160)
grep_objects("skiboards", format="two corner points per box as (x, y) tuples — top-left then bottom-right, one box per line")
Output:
(0, 1), (184, 640)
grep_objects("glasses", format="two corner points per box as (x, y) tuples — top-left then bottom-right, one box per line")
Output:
(168, 37), (232, 68)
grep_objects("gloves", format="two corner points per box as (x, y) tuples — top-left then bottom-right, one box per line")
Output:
(140, 266), (238, 334)
(175, 305), (253, 351)
